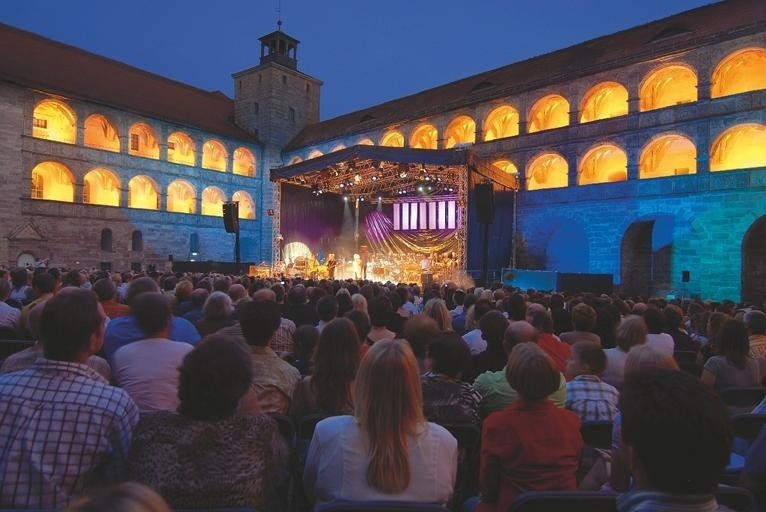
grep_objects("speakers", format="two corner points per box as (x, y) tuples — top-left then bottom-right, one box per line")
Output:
(680, 270), (690, 283)
(169, 255), (174, 262)
(475, 183), (496, 225)
(221, 203), (239, 234)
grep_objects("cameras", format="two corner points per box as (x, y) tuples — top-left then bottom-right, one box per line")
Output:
(279, 280), (286, 285)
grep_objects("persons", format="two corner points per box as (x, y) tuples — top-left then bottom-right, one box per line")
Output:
(0, 247), (764, 512)
